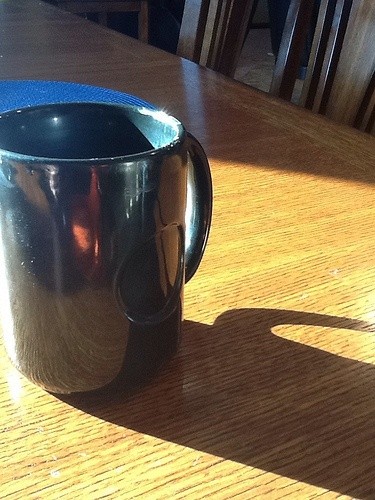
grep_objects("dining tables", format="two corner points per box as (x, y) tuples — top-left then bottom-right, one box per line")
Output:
(1, 1), (373, 500)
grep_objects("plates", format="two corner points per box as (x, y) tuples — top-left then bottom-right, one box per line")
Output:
(0, 79), (159, 117)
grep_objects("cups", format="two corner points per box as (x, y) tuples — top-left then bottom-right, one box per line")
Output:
(1, 101), (213, 394)
